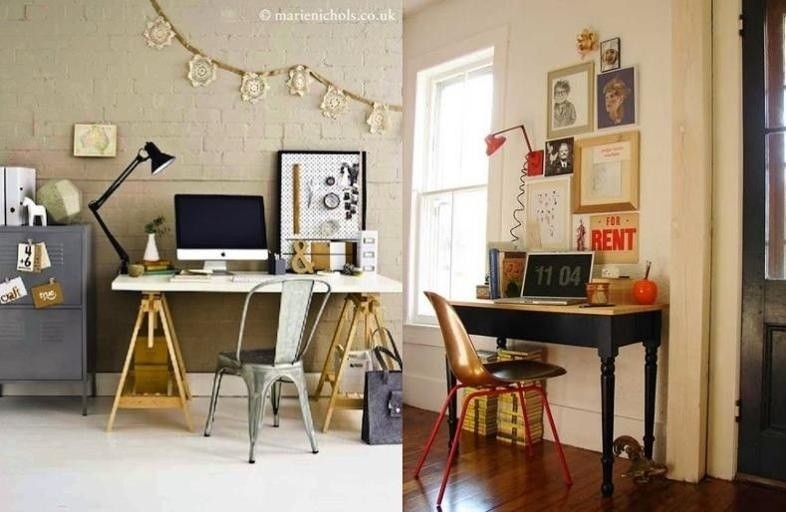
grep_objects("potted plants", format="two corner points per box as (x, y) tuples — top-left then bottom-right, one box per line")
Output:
(142, 216), (170, 263)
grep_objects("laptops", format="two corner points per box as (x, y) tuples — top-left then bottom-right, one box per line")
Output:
(494, 251), (595, 306)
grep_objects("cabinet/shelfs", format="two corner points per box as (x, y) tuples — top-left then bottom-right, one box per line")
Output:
(0, 226), (97, 419)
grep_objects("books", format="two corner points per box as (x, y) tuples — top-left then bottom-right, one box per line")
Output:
(461, 341), (546, 450)
(486, 240), (528, 299)
(136, 260), (213, 284)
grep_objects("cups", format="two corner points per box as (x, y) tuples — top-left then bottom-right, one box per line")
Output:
(584, 281), (611, 306)
(128, 263), (144, 278)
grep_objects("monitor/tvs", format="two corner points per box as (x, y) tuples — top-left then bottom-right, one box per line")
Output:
(175, 194), (269, 275)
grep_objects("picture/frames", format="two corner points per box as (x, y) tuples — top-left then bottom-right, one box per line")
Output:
(521, 33), (651, 268)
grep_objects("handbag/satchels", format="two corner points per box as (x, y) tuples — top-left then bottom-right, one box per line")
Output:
(361, 325), (402, 445)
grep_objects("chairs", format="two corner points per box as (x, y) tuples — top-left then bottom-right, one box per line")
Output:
(205, 276), (333, 467)
(414, 289), (570, 510)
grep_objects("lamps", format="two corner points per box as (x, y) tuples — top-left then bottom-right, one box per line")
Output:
(485, 122), (546, 176)
(90, 137), (175, 277)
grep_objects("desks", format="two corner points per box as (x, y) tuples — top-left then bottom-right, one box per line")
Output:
(107, 263), (405, 431)
(441, 290), (666, 499)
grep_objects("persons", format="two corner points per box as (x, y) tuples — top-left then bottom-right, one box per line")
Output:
(603, 78), (631, 126)
(602, 41), (619, 70)
(546, 140), (574, 177)
(553, 80), (576, 128)
(22, 196), (46, 227)
